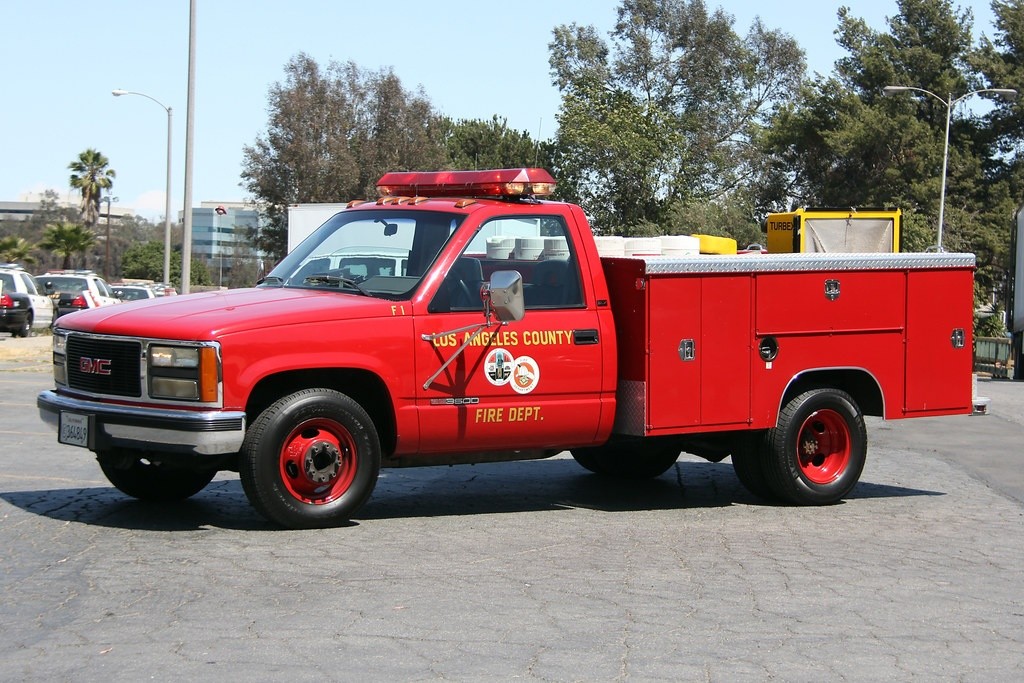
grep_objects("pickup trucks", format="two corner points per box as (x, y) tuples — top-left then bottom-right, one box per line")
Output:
(35, 168), (990, 530)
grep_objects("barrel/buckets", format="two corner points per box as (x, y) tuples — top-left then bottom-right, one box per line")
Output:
(486, 236), (700, 261)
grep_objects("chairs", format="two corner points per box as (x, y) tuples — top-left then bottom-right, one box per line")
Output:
(451, 257), (484, 305)
(531, 259), (582, 305)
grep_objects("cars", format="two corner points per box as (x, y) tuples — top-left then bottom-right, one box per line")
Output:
(107, 281), (156, 304)
(33, 270), (124, 323)
(0, 264), (59, 338)
(146, 284), (178, 299)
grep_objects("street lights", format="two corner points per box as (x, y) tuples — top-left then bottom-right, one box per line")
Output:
(111, 90), (175, 284)
(883, 83), (1017, 253)
